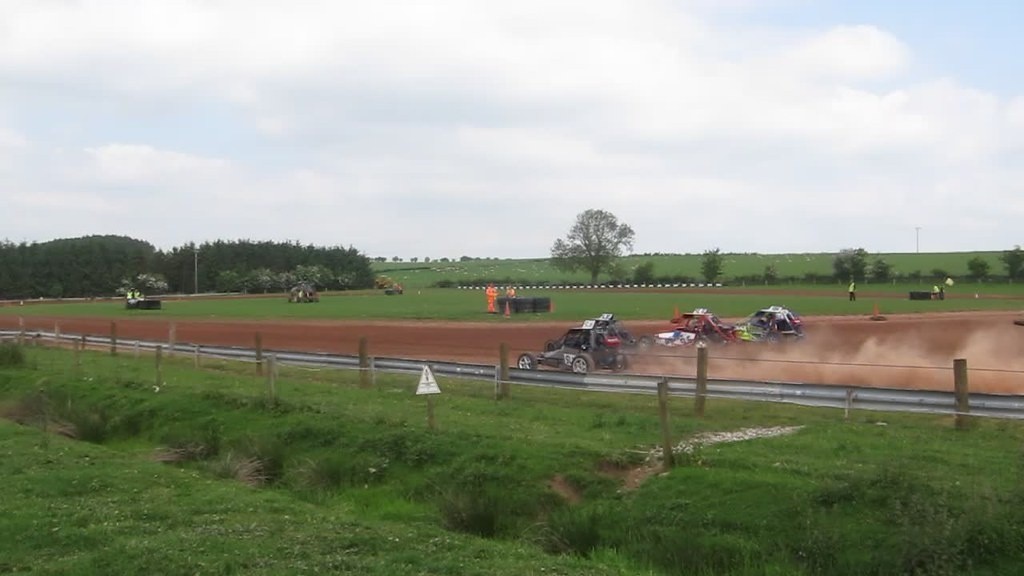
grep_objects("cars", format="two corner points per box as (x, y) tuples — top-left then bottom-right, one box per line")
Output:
(516, 313), (654, 374)
(655, 308), (740, 348)
(729, 305), (804, 347)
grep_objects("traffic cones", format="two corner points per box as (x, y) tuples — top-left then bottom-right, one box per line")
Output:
(504, 301), (511, 320)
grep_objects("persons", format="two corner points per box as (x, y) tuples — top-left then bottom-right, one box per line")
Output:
(485, 281), (500, 313)
(767, 313), (776, 330)
(506, 283), (515, 297)
(847, 279), (856, 302)
